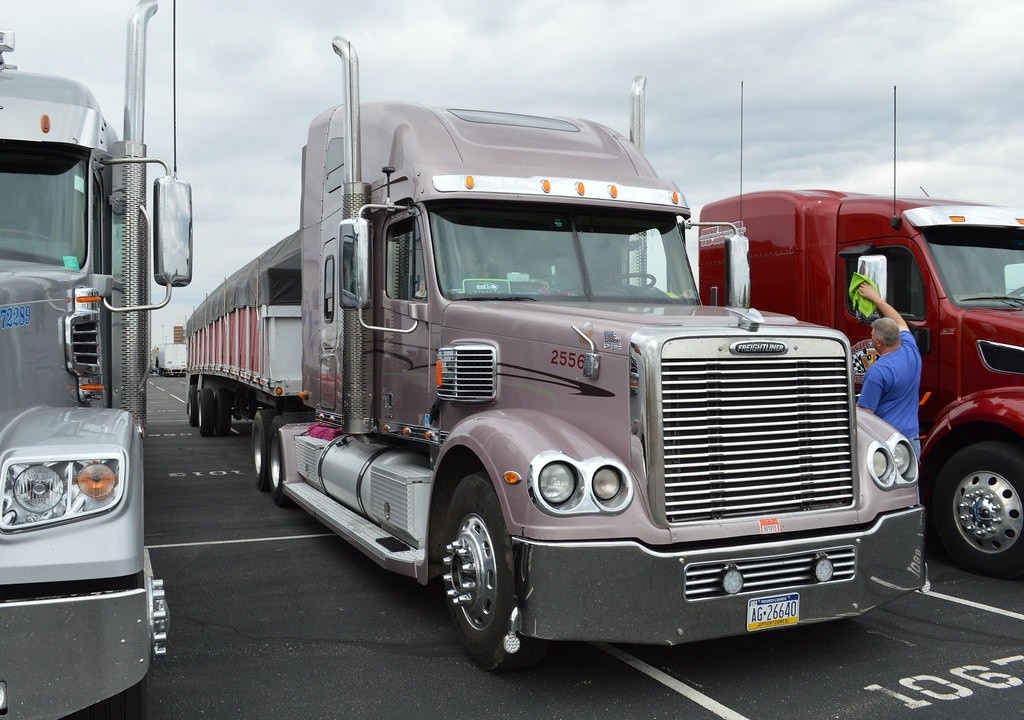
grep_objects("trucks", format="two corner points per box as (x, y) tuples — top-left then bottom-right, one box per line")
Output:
(157, 344), (186, 377)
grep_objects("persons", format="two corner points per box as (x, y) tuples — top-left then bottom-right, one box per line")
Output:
(856, 282), (924, 461)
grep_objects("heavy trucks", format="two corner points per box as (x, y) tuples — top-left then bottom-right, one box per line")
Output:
(694, 188), (1024, 579)
(0, 0), (193, 719)
(185, 35), (928, 672)
